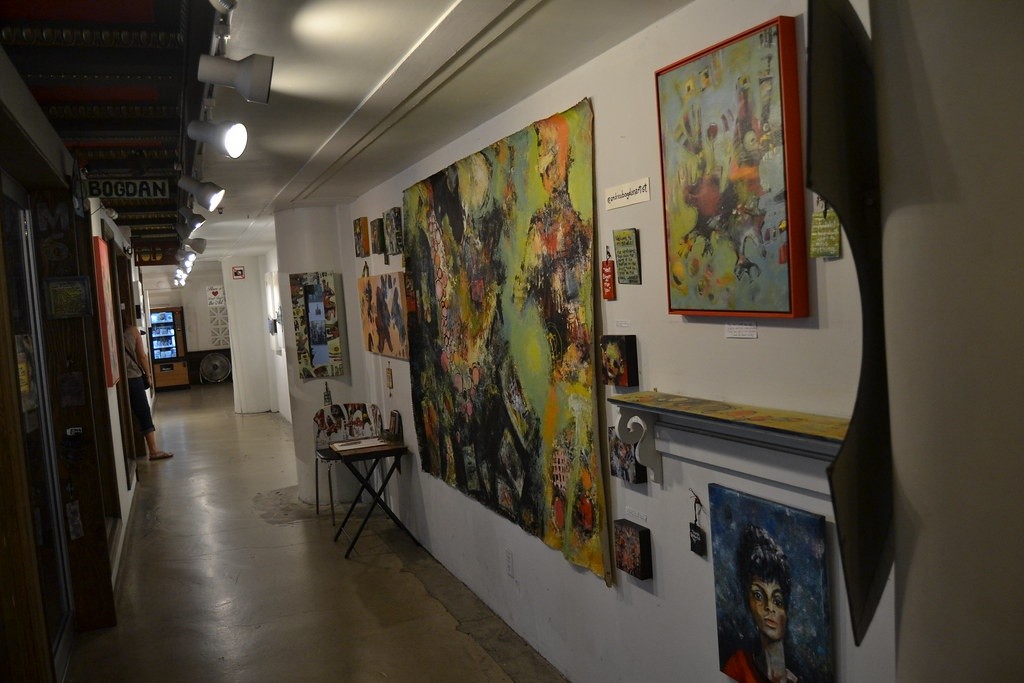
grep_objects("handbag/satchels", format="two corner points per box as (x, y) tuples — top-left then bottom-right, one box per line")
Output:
(141, 374), (150, 389)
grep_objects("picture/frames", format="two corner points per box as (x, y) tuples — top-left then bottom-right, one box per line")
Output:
(610, 518), (656, 581)
(608, 426), (648, 485)
(598, 333), (639, 389)
(653, 14), (813, 320)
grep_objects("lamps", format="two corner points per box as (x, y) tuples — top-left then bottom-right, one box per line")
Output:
(185, 119), (247, 160)
(105, 208), (119, 220)
(123, 247), (132, 254)
(196, 52), (274, 105)
(174, 175), (225, 287)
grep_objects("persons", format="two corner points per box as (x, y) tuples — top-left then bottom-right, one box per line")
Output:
(120, 306), (175, 461)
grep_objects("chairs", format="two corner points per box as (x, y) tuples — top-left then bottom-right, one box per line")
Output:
(312, 403), (385, 527)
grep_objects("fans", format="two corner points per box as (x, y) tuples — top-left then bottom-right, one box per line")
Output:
(199, 353), (232, 384)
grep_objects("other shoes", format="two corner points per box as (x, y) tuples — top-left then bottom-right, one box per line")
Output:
(150, 450), (174, 460)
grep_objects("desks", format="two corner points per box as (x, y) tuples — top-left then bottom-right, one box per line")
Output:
(327, 435), (422, 560)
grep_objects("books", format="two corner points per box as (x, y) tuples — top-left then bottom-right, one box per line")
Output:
(389, 410), (399, 435)
